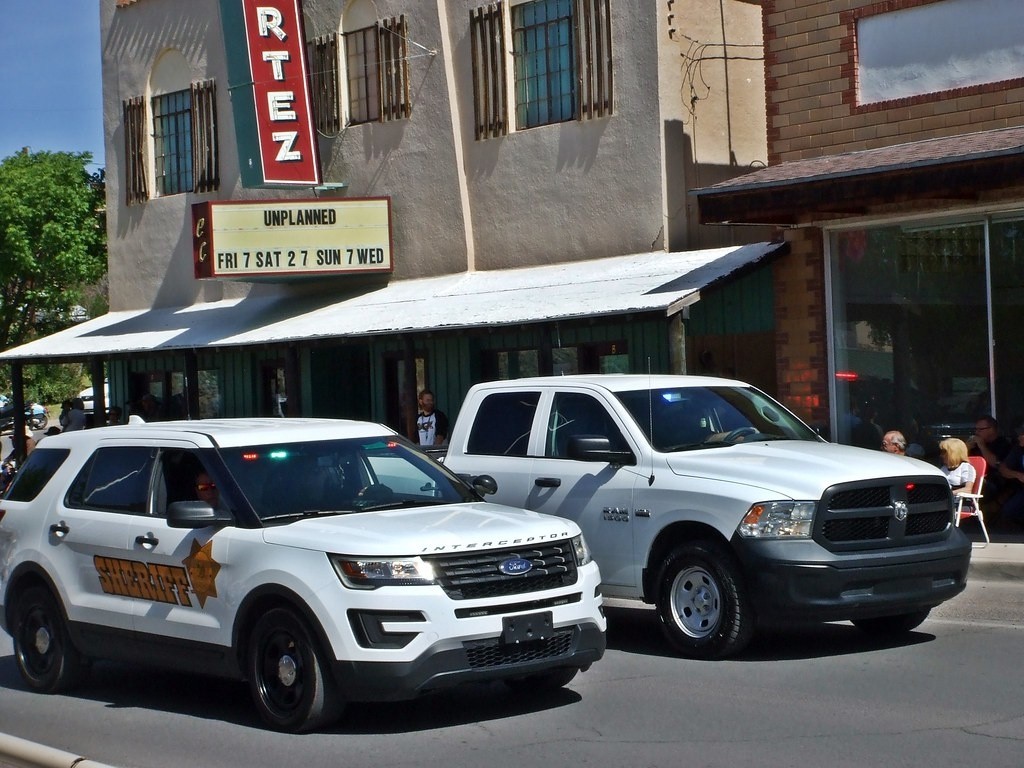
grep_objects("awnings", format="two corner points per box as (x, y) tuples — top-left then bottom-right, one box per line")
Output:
(0, 238), (784, 459)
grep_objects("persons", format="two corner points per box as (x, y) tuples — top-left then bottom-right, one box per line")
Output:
(59, 399), (72, 432)
(663, 401), (760, 446)
(966, 416), (1012, 505)
(61, 398), (86, 431)
(863, 407), (883, 437)
(1000, 418), (1024, 521)
(408, 388), (449, 446)
(4, 425), (36, 467)
(881, 430), (908, 457)
(108, 407), (123, 425)
(195, 471), (231, 518)
(285, 456), (343, 513)
(938, 438), (976, 513)
(83, 415), (95, 429)
(126, 390), (189, 422)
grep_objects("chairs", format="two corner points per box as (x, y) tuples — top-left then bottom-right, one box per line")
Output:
(951, 456), (990, 548)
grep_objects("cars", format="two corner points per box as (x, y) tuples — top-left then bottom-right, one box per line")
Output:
(77, 377), (110, 420)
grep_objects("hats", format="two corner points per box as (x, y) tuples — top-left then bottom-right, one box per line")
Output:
(44, 426), (61, 436)
(141, 394), (156, 402)
(9, 425), (33, 439)
(61, 399), (73, 409)
(1014, 422), (1024, 434)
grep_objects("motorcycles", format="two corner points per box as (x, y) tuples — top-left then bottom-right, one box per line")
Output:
(0, 395), (50, 430)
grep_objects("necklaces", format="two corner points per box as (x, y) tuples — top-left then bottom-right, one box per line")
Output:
(422, 414), (431, 429)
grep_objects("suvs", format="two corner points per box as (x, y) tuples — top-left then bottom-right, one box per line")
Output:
(0, 418), (607, 730)
(437, 372), (971, 657)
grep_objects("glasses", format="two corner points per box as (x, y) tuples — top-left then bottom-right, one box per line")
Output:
(939, 449), (947, 455)
(194, 482), (216, 492)
(973, 427), (993, 432)
(108, 412), (117, 416)
(881, 442), (894, 447)
